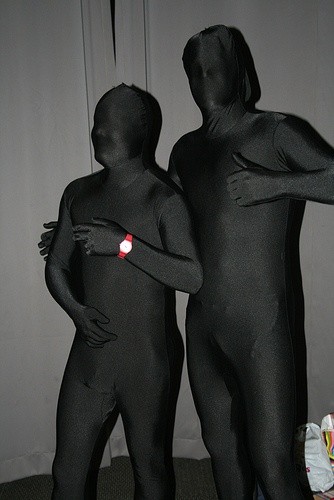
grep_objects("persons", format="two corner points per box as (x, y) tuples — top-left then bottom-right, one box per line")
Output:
(27, 82), (205, 498)
(35, 26), (334, 499)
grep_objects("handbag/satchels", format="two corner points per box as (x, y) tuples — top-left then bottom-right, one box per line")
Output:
(320, 414), (334, 468)
(304, 421), (332, 491)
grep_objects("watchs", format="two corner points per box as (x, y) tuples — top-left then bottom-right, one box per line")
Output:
(114, 230), (134, 258)
